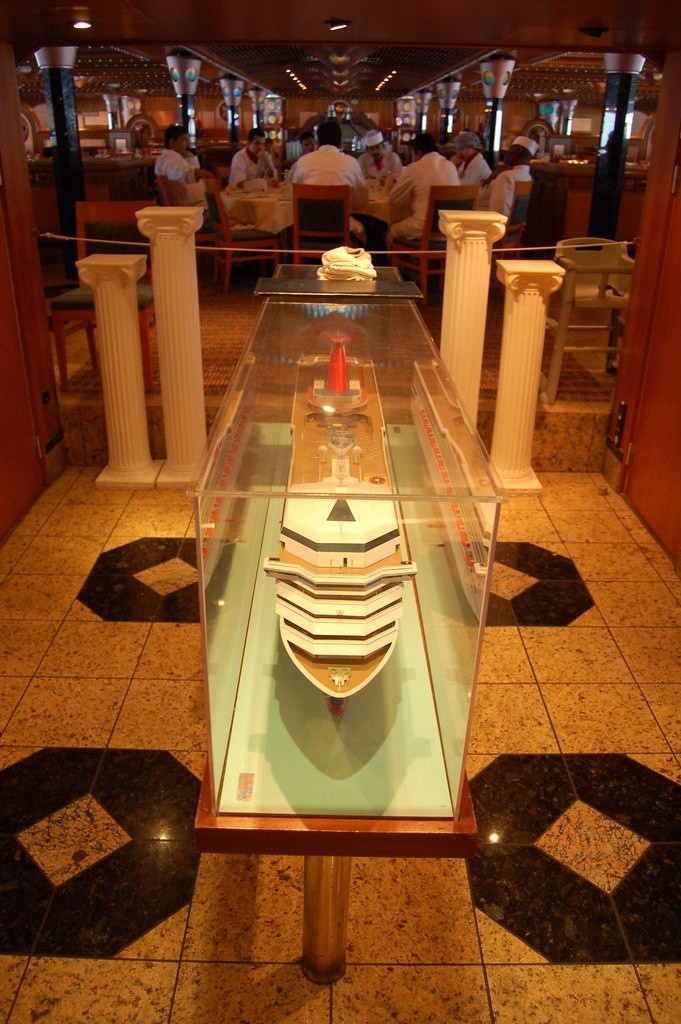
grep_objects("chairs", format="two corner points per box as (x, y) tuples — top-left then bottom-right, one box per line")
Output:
(290, 182), (361, 264)
(208, 176), (282, 295)
(215, 160), (233, 187)
(541, 233), (637, 402)
(153, 171), (216, 257)
(389, 182), (482, 305)
(491, 176), (540, 286)
(49, 195), (163, 394)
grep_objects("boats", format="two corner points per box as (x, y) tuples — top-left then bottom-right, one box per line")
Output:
(262, 332), (418, 717)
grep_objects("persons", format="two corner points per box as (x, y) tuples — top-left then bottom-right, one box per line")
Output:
(387, 133), (460, 248)
(358, 129), (402, 178)
(478, 136), (539, 216)
(454, 132), (492, 184)
(291, 132), (317, 172)
(229, 128), (277, 188)
(293, 121), (367, 245)
(154, 125), (213, 183)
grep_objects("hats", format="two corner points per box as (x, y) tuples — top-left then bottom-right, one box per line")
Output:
(363, 130), (383, 147)
(513, 135), (538, 156)
(455, 132), (481, 150)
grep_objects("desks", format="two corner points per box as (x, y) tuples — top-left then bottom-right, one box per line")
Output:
(28, 154), (155, 193)
(221, 178), (499, 242)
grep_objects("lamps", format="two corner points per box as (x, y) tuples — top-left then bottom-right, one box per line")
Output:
(327, 17), (353, 32)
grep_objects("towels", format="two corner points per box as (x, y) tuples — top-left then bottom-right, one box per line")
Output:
(316, 246), (378, 282)
